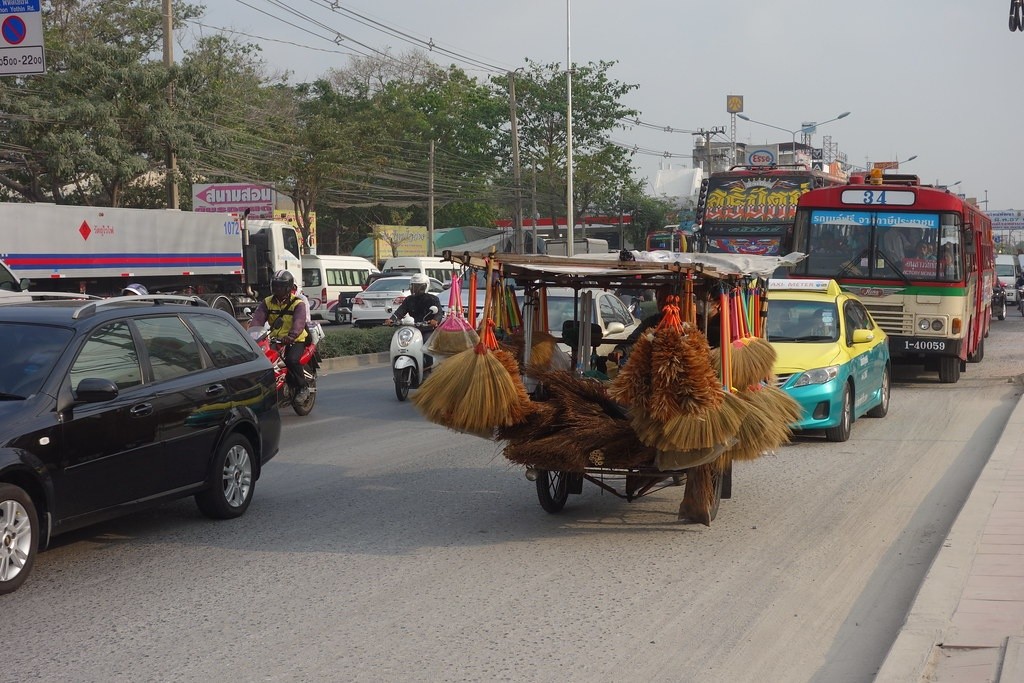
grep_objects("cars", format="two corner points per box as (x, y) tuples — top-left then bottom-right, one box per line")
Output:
(746, 278), (893, 443)
(435, 261), (657, 396)
(991, 273), (1008, 320)
(349, 274), (445, 329)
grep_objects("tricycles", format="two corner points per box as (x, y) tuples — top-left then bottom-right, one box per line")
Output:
(439, 247), (808, 522)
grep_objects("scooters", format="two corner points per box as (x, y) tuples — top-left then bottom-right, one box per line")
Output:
(382, 304), (440, 402)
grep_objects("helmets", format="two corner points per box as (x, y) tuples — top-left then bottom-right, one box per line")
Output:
(270, 269), (294, 293)
(410, 273), (430, 294)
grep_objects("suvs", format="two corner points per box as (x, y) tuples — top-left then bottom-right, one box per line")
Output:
(0, 289), (283, 597)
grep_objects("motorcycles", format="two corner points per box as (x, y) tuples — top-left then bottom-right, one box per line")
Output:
(1015, 285), (1024, 317)
(245, 321), (325, 417)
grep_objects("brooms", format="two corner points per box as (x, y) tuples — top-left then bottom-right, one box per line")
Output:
(719, 286), (808, 474)
(482, 272), (749, 479)
(408, 246), (516, 432)
(710, 286), (776, 388)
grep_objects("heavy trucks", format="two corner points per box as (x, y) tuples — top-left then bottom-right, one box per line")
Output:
(0, 203), (312, 321)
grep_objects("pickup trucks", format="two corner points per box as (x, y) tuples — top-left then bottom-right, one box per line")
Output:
(336, 270), (416, 327)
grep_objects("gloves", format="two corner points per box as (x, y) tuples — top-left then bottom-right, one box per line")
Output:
(428, 320), (438, 327)
(281, 334), (296, 347)
(385, 320), (393, 327)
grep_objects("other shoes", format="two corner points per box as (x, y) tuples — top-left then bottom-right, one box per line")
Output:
(423, 371), (430, 380)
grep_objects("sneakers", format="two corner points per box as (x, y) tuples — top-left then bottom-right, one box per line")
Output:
(294, 386), (310, 405)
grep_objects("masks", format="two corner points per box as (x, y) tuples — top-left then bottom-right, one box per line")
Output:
(695, 300), (717, 314)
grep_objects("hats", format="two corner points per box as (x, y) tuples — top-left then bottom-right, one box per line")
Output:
(122, 283), (149, 301)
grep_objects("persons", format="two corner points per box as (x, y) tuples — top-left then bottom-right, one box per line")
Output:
(247, 269), (311, 406)
(289, 283), (311, 328)
(808, 308), (834, 336)
(613, 283), (677, 363)
(384, 272), (443, 373)
(883, 226), (962, 276)
(122, 283), (149, 302)
(811, 231), (849, 257)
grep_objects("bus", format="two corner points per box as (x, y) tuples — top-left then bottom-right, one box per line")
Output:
(644, 164), (998, 384)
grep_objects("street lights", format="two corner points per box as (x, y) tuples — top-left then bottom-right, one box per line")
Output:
(736, 112), (853, 169)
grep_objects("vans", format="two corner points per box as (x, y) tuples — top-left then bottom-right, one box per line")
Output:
(995, 253), (1024, 304)
(0, 258), (33, 304)
(382, 256), (469, 283)
(300, 252), (382, 325)
